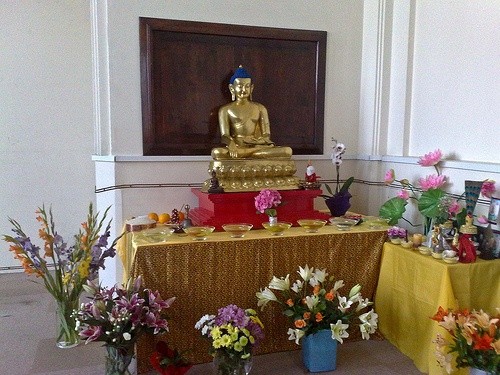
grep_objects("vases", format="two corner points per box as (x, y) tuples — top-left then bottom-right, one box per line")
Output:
(471, 367), (500, 375)
(324, 193), (352, 218)
(269, 217), (277, 226)
(105, 343), (134, 375)
(303, 329), (336, 372)
(55, 293), (80, 350)
(215, 348), (252, 375)
(391, 236), (402, 244)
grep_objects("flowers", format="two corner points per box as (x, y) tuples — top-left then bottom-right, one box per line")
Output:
(72, 274), (176, 375)
(255, 189), (282, 216)
(257, 263), (378, 344)
(379, 151), (496, 236)
(317, 140), (354, 197)
(386, 226), (407, 239)
(0, 204), (125, 341)
(431, 305), (500, 375)
(194, 304), (263, 361)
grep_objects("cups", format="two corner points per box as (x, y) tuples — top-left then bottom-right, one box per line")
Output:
(412, 234), (422, 247)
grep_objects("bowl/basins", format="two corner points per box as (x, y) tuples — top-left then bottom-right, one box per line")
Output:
(297, 219), (327, 233)
(141, 227), (175, 242)
(182, 225), (215, 241)
(401, 240), (413, 248)
(261, 221), (292, 237)
(222, 223), (253, 238)
(443, 256), (457, 264)
(417, 246), (430, 256)
(329, 216), (359, 230)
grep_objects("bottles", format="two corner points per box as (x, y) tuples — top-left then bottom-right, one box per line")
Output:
(457, 214), (478, 263)
(430, 227), (444, 259)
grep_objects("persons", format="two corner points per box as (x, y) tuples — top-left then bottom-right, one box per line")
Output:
(304, 164), (321, 185)
(200, 65), (306, 191)
(208, 170), (224, 193)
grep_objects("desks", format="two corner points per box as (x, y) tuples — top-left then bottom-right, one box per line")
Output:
(374, 245), (500, 375)
(124, 211), (402, 375)
